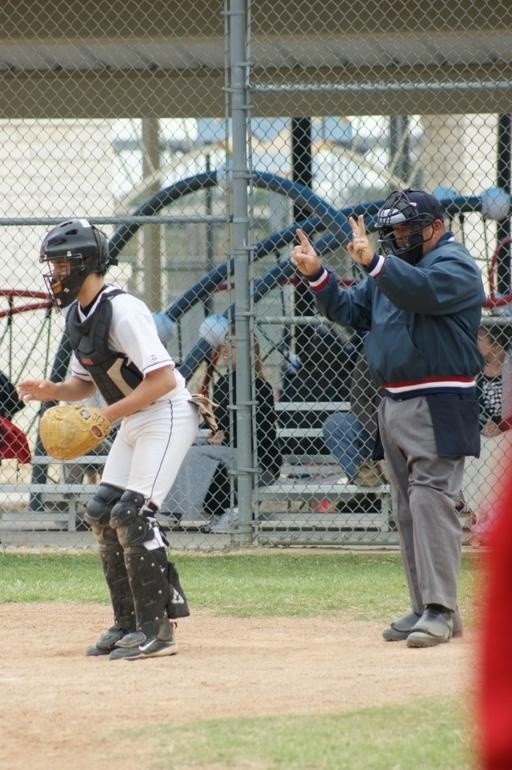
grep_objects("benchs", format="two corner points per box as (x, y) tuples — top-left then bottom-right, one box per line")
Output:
(251, 402), (471, 541)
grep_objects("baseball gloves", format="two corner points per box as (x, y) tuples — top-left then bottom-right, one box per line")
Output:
(39, 406), (112, 460)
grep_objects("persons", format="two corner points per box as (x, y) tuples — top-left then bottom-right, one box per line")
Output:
(288, 186), (484, 651)
(456, 313), (510, 546)
(15, 218), (202, 663)
(319, 334), (393, 487)
(197, 325), (284, 532)
(38, 369), (120, 526)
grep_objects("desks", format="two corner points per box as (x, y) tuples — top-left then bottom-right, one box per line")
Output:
(159, 444), (237, 521)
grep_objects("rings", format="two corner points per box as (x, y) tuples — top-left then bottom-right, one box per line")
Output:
(359, 236), (363, 244)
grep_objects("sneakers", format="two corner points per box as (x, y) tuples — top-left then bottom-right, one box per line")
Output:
(335, 492), (382, 513)
(212, 508), (239, 533)
(383, 611), (463, 640)
(407, 610), (452, 646)
(88, 637), (177, 660)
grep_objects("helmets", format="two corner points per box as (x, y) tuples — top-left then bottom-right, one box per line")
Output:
(374, 190), (443, 266)
(40, 219), (109, 307)
